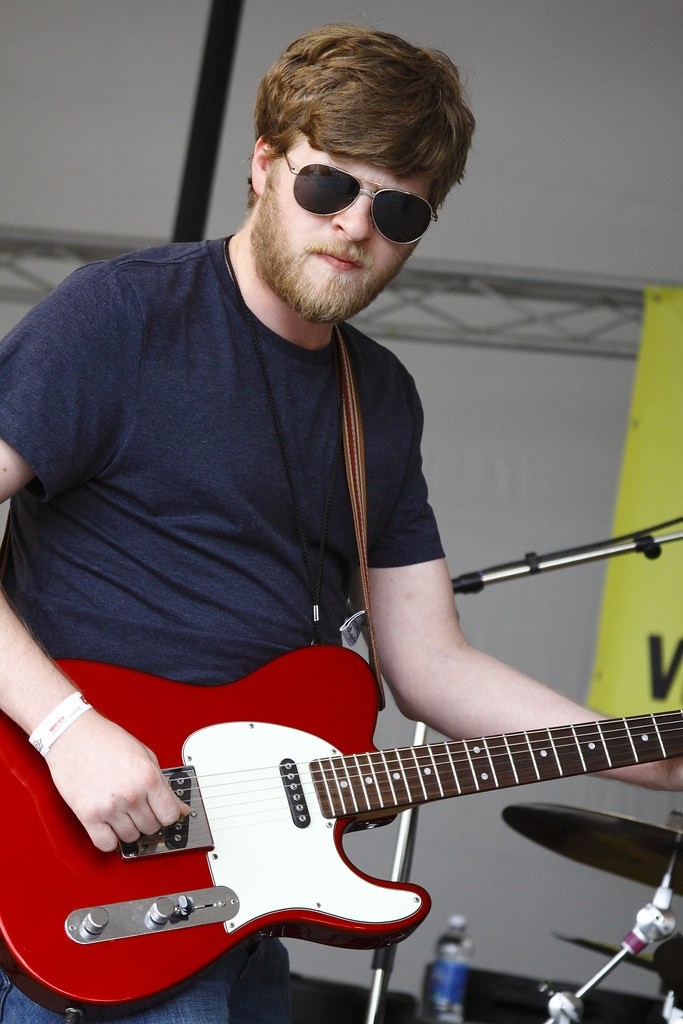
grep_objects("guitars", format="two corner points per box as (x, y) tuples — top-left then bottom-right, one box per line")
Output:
(2, 641), (683, 1024)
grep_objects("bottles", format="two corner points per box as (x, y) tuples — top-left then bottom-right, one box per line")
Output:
(427, 915), (473, 1024)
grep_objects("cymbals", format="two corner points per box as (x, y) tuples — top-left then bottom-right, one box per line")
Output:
(501, 801), (683, 896)
(552, 929), (657, 970)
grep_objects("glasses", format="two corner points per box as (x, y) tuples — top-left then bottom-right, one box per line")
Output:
(281, 149), (438, 244)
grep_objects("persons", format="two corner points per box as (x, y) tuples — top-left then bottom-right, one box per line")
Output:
(0, 26), (683, 1024)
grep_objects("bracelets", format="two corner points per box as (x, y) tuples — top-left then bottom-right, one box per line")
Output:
(28, 692), (92, 757)
